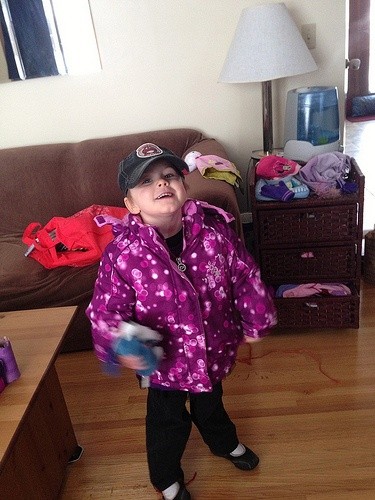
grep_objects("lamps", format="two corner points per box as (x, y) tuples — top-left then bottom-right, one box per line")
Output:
(218, 2), (318, 157)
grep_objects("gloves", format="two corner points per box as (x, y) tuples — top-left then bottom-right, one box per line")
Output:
(258, 179), (296, 202)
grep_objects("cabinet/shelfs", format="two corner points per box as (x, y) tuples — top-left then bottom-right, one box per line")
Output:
(249, 157), (364, 328)
(0, 305), (78, 500)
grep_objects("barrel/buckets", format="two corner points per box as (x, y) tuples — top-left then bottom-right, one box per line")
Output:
(283, 86), (340, 163)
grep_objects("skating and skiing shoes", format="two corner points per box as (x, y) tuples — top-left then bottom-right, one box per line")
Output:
(217, 439), (260, 470)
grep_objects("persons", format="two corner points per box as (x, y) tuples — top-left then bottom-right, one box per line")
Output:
(83, 141), (282, 500)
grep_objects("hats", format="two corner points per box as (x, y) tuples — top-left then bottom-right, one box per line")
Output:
(196, 156), (242, 189)
(116, 137), (189, 194)
(254, 154), (302, 182)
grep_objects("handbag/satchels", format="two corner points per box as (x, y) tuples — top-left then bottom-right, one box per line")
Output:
(16, 203), (133, 272)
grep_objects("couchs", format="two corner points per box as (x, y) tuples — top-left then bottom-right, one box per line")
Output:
(0, 129), (244, 353)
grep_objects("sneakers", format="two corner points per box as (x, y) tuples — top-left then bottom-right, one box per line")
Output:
(157, 466), (192, 500)
